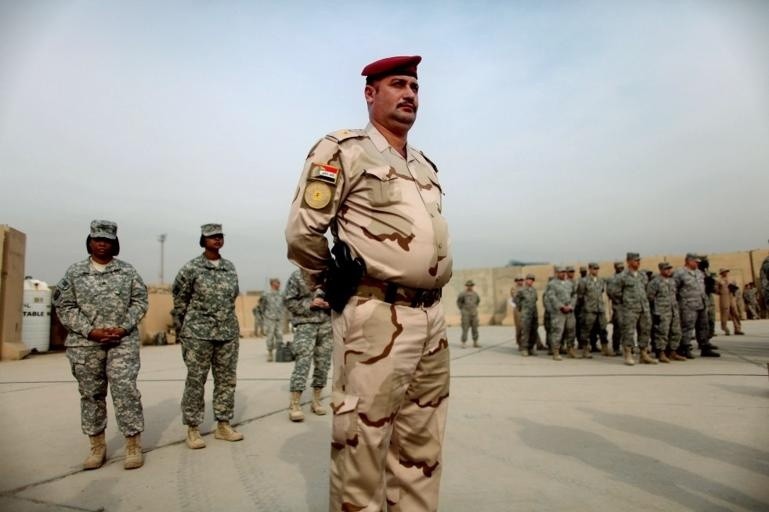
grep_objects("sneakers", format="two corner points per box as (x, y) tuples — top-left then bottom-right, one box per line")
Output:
(726, 331), (729, 336)
(735, 331), (744, 334)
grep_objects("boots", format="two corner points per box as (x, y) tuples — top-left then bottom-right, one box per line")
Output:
(624, 348), (695, 365)
(185, 424), (205, 449)
(289, 391), (305, 422)
(268, 351), (273, 361)
(698, 342), (719, 357)
(553, 346), (593, 360)
(215, 422), (243, 441)
(83, 432), (106, 469)
(591, 342), (623, 355)
(520, 343), (547, 356)
(124, 434), (144, 469)
(474, 341), (481, 348)
(462, 342), (465, 348)
(311, 387), (327, 416)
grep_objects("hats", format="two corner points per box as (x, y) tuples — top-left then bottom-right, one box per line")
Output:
(687, 253), (709, 263)
(614, 262), (624, 269)
(526, 274), (535, 279)
(270, 277), (281, 283)
(554, 263), (600, 272)
(658, 262), (672, 268)
(719, 268), (729, 274)
(514, 277), (524, 281)
(201, 223), (225, 237)
(627, 253), (642, 260)
(465, 280), (474, 286)
(362, 56), (422, 75)
(89, 220), (117, 240)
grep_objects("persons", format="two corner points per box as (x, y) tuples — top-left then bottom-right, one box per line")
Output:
(252, 299), (267, 337)
(509, 250), (769, 365)
(260, 277), (288, 361)
(457, 280), (481, 348)
(281, 266), (335, 421)
(169, 306), (181, 345)
(284, 55), (453, 511)
(172, 223), (244, 450)
(54, 218), (147, 474)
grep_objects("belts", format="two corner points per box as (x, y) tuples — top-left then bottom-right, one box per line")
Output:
(355, 278), (442, 309)
